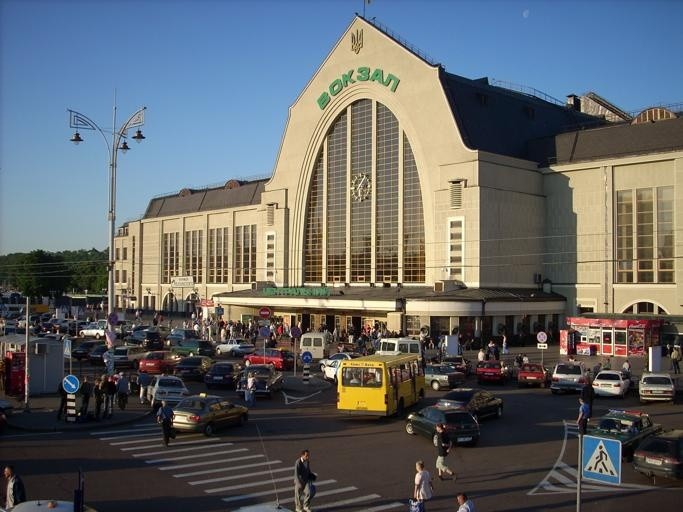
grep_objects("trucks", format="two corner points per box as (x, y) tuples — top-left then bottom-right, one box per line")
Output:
(374, 337), (422, 361)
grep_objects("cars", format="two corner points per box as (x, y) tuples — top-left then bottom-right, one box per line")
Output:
(170, 393), (248, 438)
(638, 370), (675, 406)
(424, 361), (464, 391)
(585, 408), (662, 462)
(404, 405), (480, 447)
(517, 363), (549, 388)
(435, 387), (504, 424)
(439, 354), (472, 378)
(476, 360), (513, 386)
(591, 370), (630, 400)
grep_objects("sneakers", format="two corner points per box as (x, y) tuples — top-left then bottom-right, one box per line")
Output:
(452, 472), (457, 483)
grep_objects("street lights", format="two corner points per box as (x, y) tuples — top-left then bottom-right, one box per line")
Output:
(66, 88), (147, 405)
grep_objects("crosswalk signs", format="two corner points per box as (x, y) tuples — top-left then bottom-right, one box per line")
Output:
(581, 434), (622, 486)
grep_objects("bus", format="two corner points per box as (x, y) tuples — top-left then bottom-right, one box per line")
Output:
(336, 350), (427, 416)
(661, 332), (683, 350)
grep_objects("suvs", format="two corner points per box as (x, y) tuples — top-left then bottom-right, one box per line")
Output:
(550, 358), (590, 396)
(631, 428), (683, 482)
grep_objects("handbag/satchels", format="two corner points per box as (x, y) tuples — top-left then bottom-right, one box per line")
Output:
(408, 498), (425, 512)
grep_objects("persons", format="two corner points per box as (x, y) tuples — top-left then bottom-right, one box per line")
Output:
(435, 423), (457, 482)
(419, 327), (529, 367)
(568, 350), (648, 378)
(667, 344), (681, 374)
(3, 465), (26, 511)
(581, 376), (594, 421)
(156, 400), (174, 448)
(455, 492), (475, 512)
(293, 450), (317, 512)
(56, 369), (147, 422)
(576, 397), (590, 434)
(413, 461), (434, 512)
(247, 370), (257, 408)
